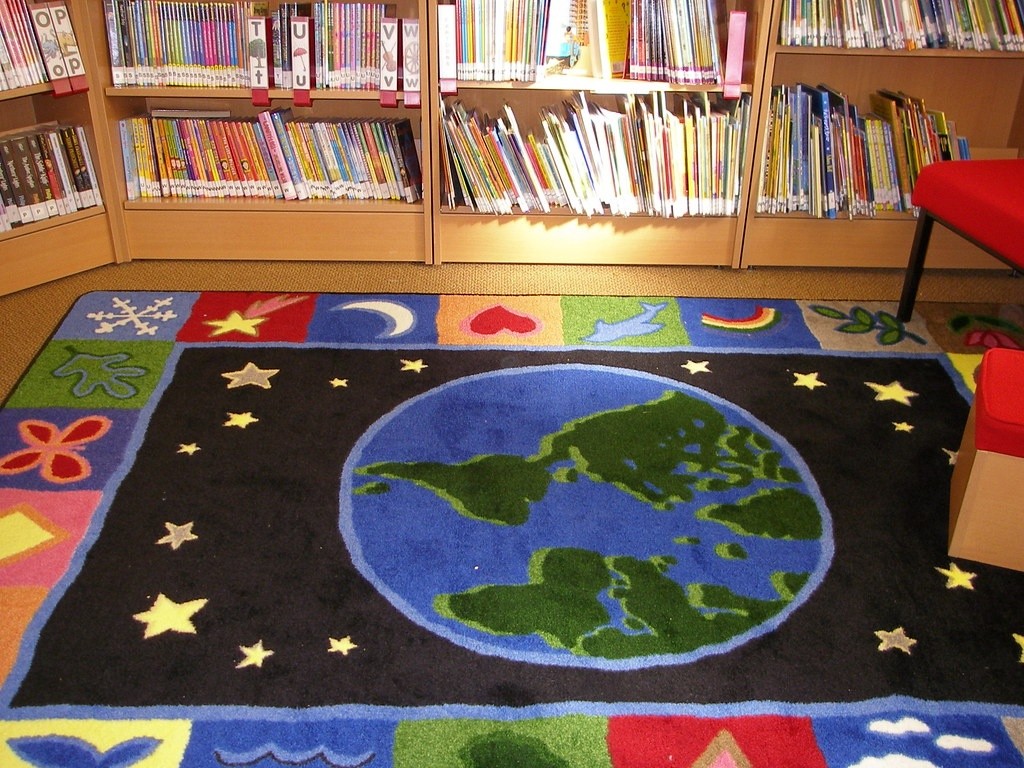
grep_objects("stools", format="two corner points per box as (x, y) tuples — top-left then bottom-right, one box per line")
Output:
(896, 155), (1024, 322)
(948, 347), (1024, 570)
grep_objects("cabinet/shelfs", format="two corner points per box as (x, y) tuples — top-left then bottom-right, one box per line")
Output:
(0, 0), (1024, 291)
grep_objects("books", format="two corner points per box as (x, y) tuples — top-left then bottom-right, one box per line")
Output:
(0, 0), (1024, 235)
